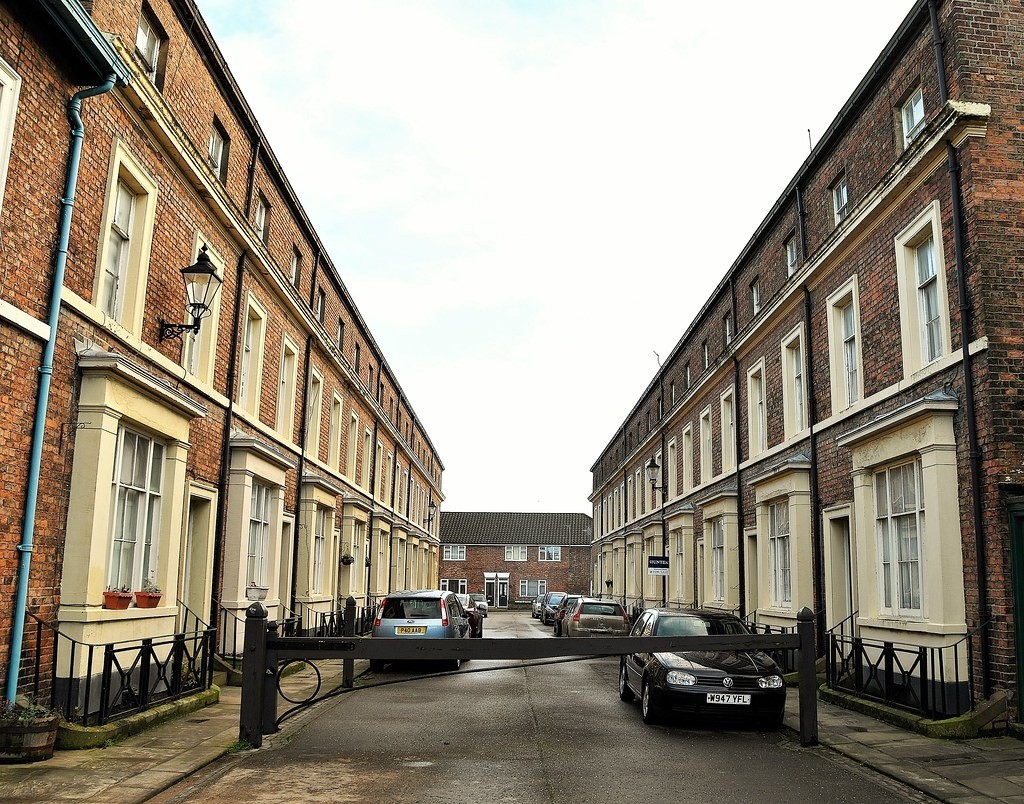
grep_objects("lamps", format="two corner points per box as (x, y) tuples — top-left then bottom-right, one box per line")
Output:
(158, 243), (224, 343)
(645, 454), (668, 495)
(423, 501), (437, 523)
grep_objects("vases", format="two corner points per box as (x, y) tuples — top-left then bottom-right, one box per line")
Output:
(246, 586), (270, 601)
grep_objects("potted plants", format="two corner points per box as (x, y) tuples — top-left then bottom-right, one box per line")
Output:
(340, 554), (354, 565)
(103, 584), (133, 610)
(0, 694), (64, 763)
(134, 569), (163, 608)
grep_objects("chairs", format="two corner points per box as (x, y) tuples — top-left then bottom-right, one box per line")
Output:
(400, 603), (411, 613)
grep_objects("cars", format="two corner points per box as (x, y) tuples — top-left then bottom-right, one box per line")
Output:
(531, 595), (546, 618)
(453, 593), (483, 638)
(470, 593), (491, 617)
(561, 597), (631, 638)
(371, 589), (472, 671)
(540, 591), (570, 625)
(618, 607), (787, 725)
(553, 595), (594, 636)
